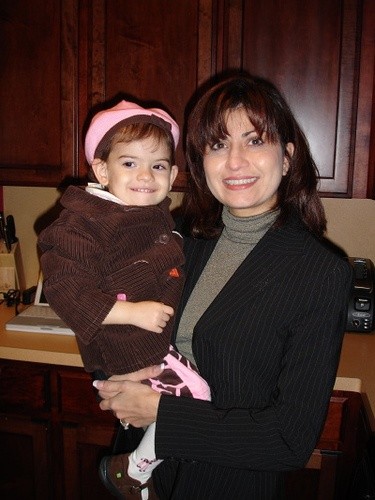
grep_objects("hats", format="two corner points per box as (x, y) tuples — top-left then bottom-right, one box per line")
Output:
(83, 100), (181, 168)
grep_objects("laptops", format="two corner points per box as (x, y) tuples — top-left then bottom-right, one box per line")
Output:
(6, 267), (79, 338)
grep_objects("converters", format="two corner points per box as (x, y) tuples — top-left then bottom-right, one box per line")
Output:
(22, 284), (36, 304)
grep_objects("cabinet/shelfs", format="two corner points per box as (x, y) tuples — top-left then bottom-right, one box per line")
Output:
(0, 0), (375, 200)
(0, 296), (375, 499)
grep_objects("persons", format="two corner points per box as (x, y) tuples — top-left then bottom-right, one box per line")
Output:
(91, 75), (356, 500)
(36, 101), (213, 500)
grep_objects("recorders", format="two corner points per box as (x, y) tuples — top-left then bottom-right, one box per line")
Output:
(344, 257), (375, 332)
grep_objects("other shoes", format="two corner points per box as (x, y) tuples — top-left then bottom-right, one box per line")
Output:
(97, 453), (161, 500)
